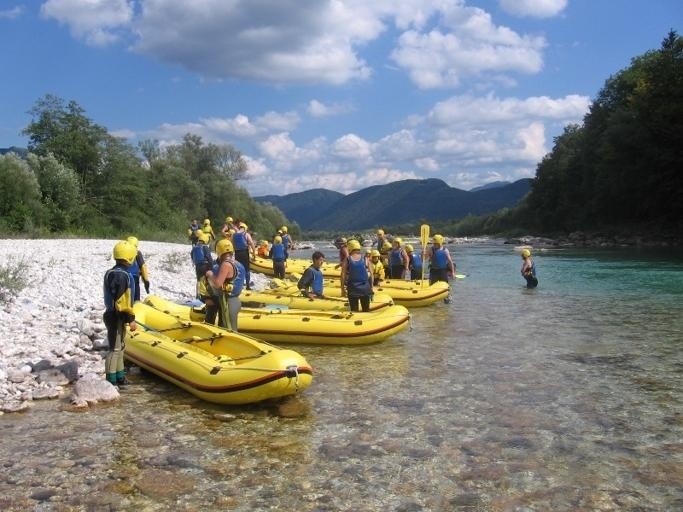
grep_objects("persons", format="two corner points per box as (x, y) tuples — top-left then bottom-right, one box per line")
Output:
(520, 248), (539, 289)
(103, 240), (138, 387)
(187, 216), (457, 333)
(339, 238), (374, 313)
(125, 235), (151, 302)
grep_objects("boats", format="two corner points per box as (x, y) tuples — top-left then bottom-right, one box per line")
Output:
(123, 300), (314, 407)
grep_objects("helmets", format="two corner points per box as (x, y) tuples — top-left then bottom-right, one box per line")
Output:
(382, 243), (392, 249)
(377, 229), (383, 237)
(238, 223), (247, 231)
(281, 227), (287, 233)
(394, 238), (401, 247)
(226, 217), (233, 222)
(198, 234), (208, 244)
(113, 240), (137, 265)
(432, 235), (443, 246)
(334, 237), (344, 243)
(189, 307), (206, 322)
(347, 239), (360, 254)
(371, 250), (380, 257)
(366, 250), (370, 254)
(203, 219), (210, 225)
(194, 229), (202, 237)
(521, 249), (530, 257)
(215, 239), (233, 259)
(405, 245), (413, 253)
(126, 237), (138, 247)
(273, 236), (281, 244)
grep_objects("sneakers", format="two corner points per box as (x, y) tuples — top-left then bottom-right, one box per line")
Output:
(116, 377), (129, 385)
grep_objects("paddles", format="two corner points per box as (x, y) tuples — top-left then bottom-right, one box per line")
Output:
(421, 225), (430, 288)
